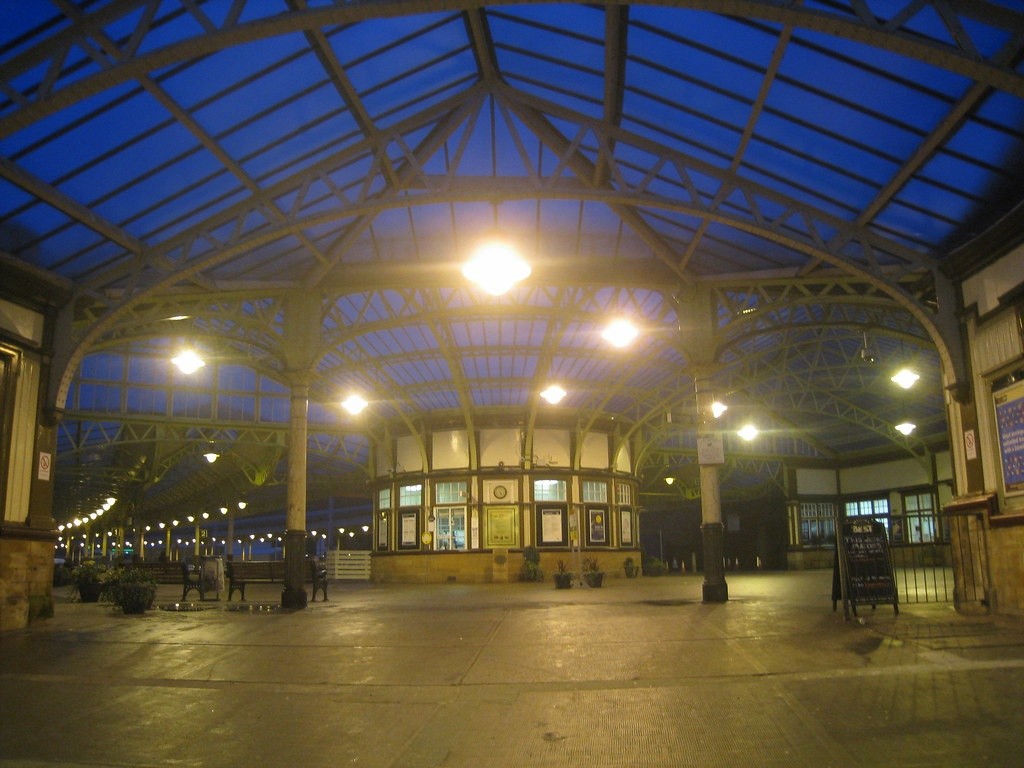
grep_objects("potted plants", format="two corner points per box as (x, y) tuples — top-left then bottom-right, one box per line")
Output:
(112, 566), (156, 614)
(582, 554), (605, 587)
(522, 545), (543, 581)
(552, 557), (571, 588)
(624, 557), (638, 577)
(72, 560), (108, 602)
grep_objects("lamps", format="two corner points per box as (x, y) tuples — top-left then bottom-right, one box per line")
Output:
(203, 442), (221, 463)
(664, 469), (676, 485)
(540, 358), (567, 404)
(895, 399), (916, 435)
(599, 285), (639, 346)
(341, 371), (368, 414)
(461, 201), (532, 296)
(891, 340), (921, 389)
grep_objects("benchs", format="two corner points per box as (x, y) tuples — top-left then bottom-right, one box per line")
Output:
(226, 559), (327, 601)
(114, 561), (203, 601)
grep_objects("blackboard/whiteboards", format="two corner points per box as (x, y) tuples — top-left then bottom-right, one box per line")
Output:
(841, 521), (900, 605)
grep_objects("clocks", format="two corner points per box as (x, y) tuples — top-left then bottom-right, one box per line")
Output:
(494, 486), (507, 499)
